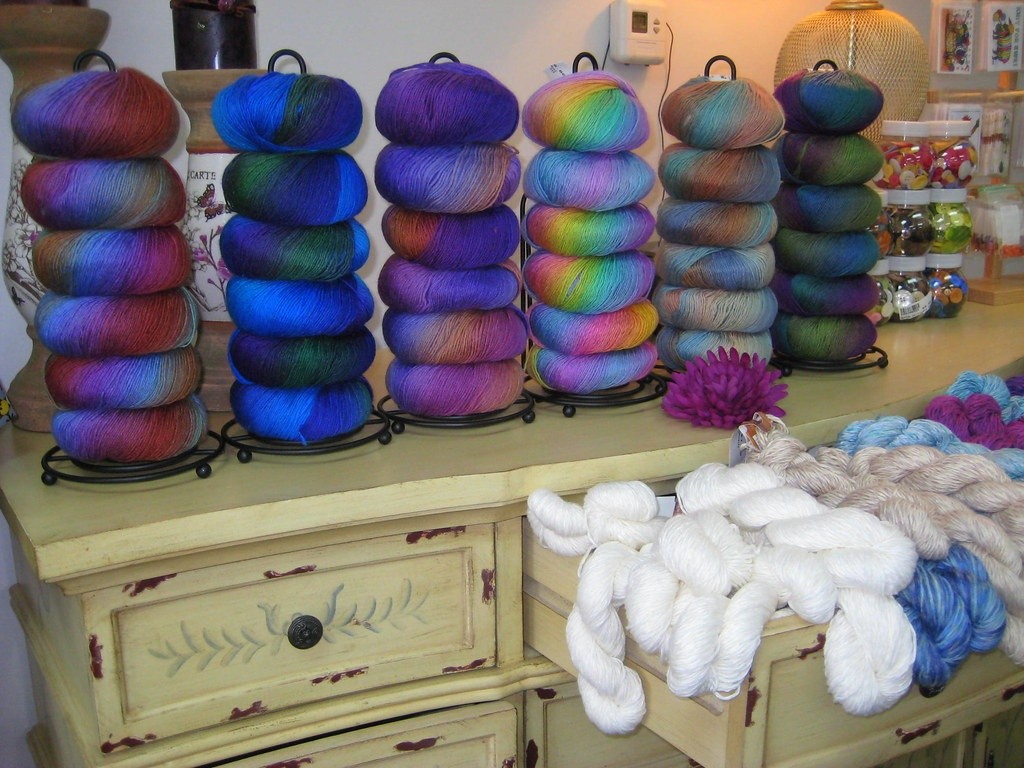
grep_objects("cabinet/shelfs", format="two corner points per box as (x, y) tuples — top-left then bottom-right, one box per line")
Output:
(3, 274), (1024, 768)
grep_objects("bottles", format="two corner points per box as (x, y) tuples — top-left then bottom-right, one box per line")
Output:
(864, 119), (979, 329)
